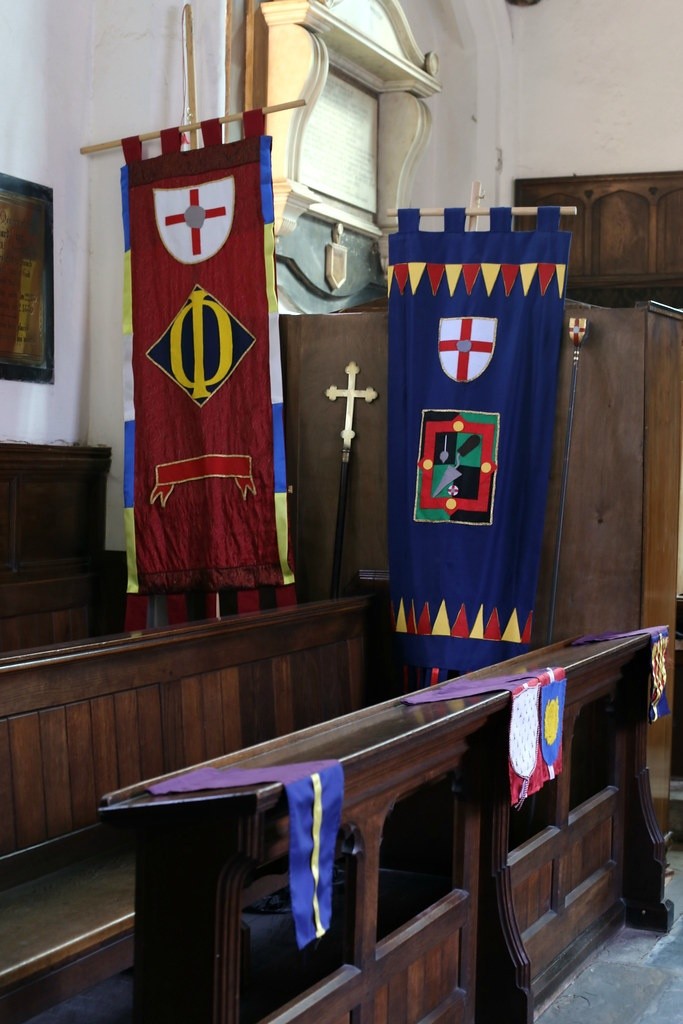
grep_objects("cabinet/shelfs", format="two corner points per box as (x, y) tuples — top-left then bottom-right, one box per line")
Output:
(512, 166), (683, 302)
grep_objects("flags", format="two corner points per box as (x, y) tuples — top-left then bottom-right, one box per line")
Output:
(385, 206), (573, 691)
(120, 107), (299, 633)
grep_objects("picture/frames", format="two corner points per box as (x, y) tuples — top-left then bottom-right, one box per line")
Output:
(0, 172), (59, 383)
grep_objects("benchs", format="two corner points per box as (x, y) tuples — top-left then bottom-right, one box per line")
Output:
(0, 598), (373, 1015)
